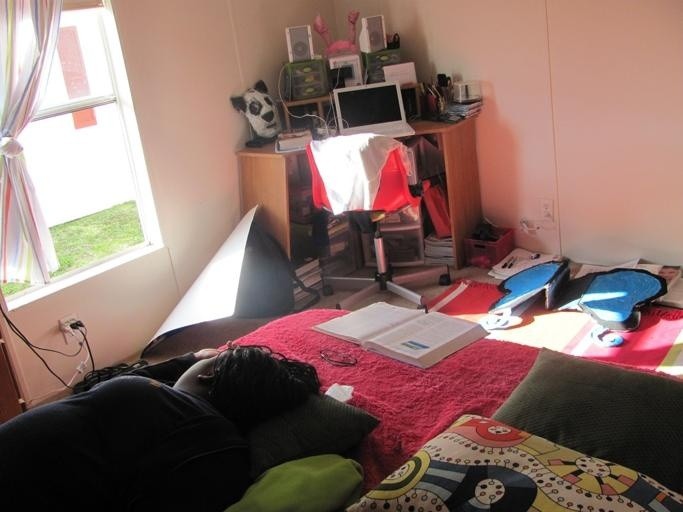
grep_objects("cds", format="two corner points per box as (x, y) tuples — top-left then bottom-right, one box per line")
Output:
(456, 95), (479, 103)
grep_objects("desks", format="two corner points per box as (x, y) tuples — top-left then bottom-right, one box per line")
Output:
(235, 84), (483, 270)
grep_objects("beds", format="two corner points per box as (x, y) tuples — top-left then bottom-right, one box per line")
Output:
(218, 308), (683, 512)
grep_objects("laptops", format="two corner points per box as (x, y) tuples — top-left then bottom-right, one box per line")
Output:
(333, 79), (415, 139)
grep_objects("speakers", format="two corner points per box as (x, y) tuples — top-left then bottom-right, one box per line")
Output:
(359, 15), (388, 53)
(285, 25), (315, 63)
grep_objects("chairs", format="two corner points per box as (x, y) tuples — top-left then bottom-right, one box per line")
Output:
(307, 132), (451, 314)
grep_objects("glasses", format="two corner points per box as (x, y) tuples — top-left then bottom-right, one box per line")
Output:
(320, 350), (357, 366)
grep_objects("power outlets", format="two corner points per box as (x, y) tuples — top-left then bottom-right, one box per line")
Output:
(59, 313), (82, 344)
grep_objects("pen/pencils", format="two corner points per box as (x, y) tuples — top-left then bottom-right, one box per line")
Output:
(501, 256), (517, 268)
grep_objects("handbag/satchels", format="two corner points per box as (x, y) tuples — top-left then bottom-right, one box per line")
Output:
(421, 178), (453, 239)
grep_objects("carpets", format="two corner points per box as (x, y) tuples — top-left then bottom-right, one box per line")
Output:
(425, 278), (683, 379)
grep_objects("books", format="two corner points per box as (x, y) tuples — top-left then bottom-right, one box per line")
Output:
(277, 127), (314, 152)
(448, 100), (481, 119)
(424, 232), (455, 267)
(310, 301), (491, 370)
(292, 255), (323, 303)
(636, 257), (682, 302)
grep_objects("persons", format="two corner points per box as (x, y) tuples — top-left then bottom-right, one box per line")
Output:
(659, 265), (680, 281)
(0, 340), (281, 512)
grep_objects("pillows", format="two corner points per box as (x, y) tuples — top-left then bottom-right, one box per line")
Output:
(250, 393), (381, 479)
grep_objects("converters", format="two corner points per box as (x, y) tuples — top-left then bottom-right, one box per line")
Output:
(317, 128), (339, 136)
